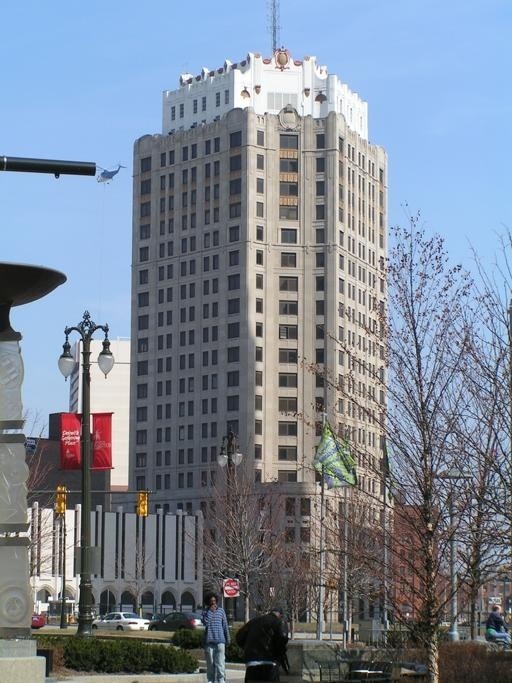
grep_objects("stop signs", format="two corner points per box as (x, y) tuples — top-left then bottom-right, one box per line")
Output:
(223, 578), (239, 598)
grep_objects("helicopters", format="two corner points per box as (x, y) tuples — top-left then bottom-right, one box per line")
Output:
(96, 162), (126, 184)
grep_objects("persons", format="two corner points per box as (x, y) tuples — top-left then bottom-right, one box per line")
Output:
(485, 604), (512, 650)
(200, 592), (231, 683)
(236, 607), (288, 683)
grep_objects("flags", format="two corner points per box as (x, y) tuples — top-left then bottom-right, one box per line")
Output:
(311, 420), (358, 490)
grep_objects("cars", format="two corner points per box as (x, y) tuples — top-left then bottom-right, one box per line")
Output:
(92, 612), (207, 632)
(32, 613), (46, 628)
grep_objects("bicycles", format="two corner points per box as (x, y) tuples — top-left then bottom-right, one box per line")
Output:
(494, 626), (512, 649)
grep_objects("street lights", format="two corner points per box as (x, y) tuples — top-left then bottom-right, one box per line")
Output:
(216, 429), (242, 626)
(437, 466), (473, 643)
(57, 309), (114, 636)
